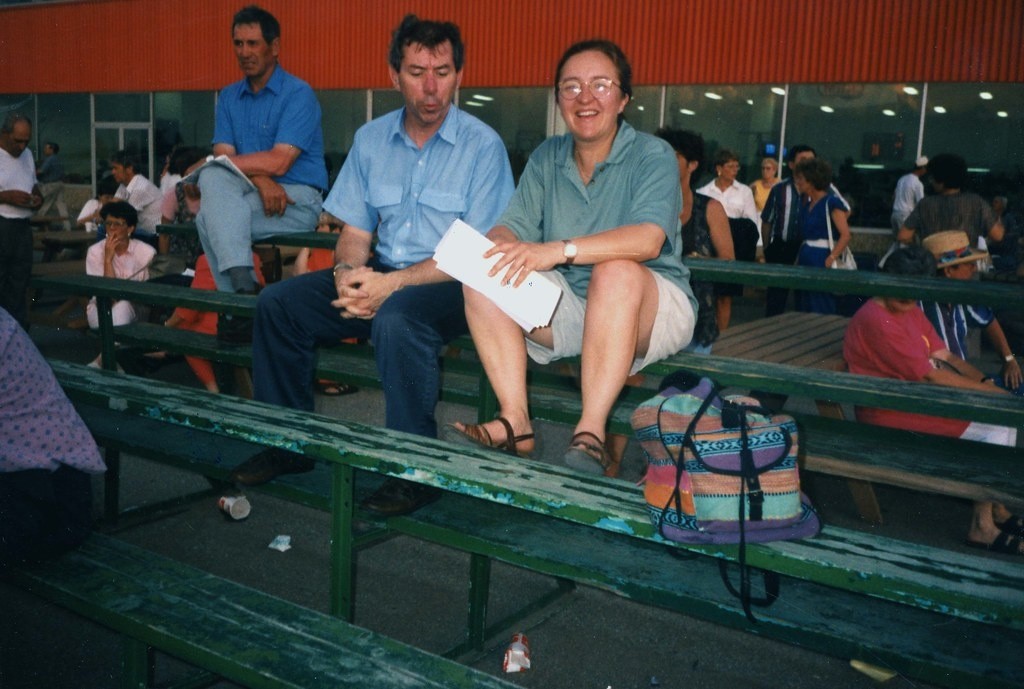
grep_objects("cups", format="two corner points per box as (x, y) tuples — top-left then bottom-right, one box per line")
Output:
(507, 633), (530, 657)
(218, 495), (250, 518)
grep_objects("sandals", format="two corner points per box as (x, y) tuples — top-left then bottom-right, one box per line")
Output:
(446, 417), (534, 457)
(564, 432), (611, 476)
(324, 382), (358, 394)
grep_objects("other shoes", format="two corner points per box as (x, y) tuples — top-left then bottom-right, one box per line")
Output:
(228, 281), (264, 332)
(216, 313), (261, 347)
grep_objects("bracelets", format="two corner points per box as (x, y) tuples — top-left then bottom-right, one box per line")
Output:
(333, 264), (354, 277)
(1002, 354), (1014, 361)
(830, 254), (834, 259)
(981, 376), (994, 384)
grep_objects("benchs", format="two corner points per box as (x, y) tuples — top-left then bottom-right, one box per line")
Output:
(0, 215), (1024, 689)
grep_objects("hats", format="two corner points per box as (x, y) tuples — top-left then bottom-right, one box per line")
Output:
(922, 231), (987, 269)
(915, 156), (928, 166)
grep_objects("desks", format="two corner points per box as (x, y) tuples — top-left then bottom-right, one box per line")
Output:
(33, 254), (87, 332)
(34, 230), (104, 255)
(683, 312), (884, 529)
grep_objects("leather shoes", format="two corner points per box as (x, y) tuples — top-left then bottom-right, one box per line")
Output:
(366, 477), (441, 514)
(235, 448), (314, 485)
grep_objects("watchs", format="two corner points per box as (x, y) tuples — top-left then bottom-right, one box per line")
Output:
(562, 239), (577, 265)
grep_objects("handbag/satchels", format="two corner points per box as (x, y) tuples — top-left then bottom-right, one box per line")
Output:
(831, 245), (858, 290)
(629, 371), (821, 546)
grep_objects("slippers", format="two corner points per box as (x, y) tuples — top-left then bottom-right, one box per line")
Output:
(992, 513), (1024, 534)
(963, 529), (1024, 554)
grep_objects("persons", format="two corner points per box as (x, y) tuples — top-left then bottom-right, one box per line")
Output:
(693, 149), (758, 331)
(841, 152), (1024, 556)
(748, 158), (782, 262)
(0, 109), (44, 327)
(0, 305), (108, 578)
(440, 38), (699, 477)
(760, 145), (852, 315)
(164, 254), (267, 395)
(233, 10), (515, 516)
(159, 145), (202, 188)
(158, 148), (213, 270)
(108, 149), (163, 255)
(37, 142), (71, 230)
(292, 212), (373, 395)
(85, 200), (158, 373)
(77, 174), (120, 247)
(603, 127), (735, 475)
(196, 4), (328, 334)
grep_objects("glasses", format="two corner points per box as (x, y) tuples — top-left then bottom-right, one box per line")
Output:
(103, 221), (129, 228)
(557, 77), (625, 101)
